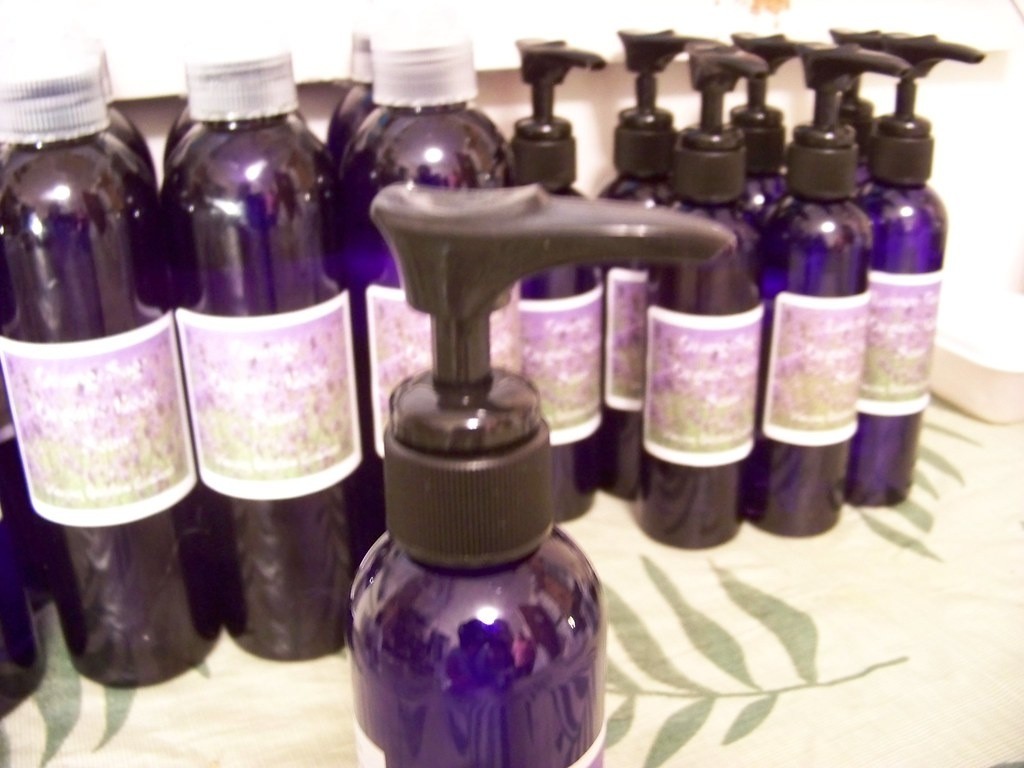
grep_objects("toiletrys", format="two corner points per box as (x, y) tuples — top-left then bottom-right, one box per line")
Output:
(0, 26), (986, 768)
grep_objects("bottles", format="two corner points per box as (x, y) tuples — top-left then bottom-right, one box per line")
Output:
(0, 21), (519, 717)
